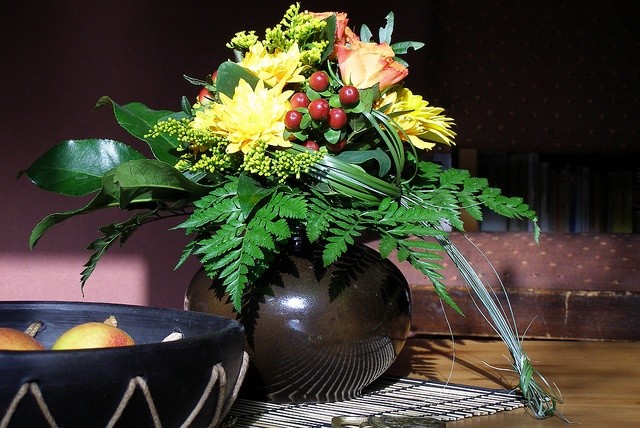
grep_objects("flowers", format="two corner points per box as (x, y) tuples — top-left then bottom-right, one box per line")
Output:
(15, 0), (542, 323)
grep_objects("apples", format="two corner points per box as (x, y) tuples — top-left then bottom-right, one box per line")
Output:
(1, 328), (50, 353)
(53, 321), (136, 349)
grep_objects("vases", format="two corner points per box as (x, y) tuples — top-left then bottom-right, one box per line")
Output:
(185, 227), (413, 403)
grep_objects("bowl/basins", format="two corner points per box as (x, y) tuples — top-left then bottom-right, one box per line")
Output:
(0, 301), (249, 428)
